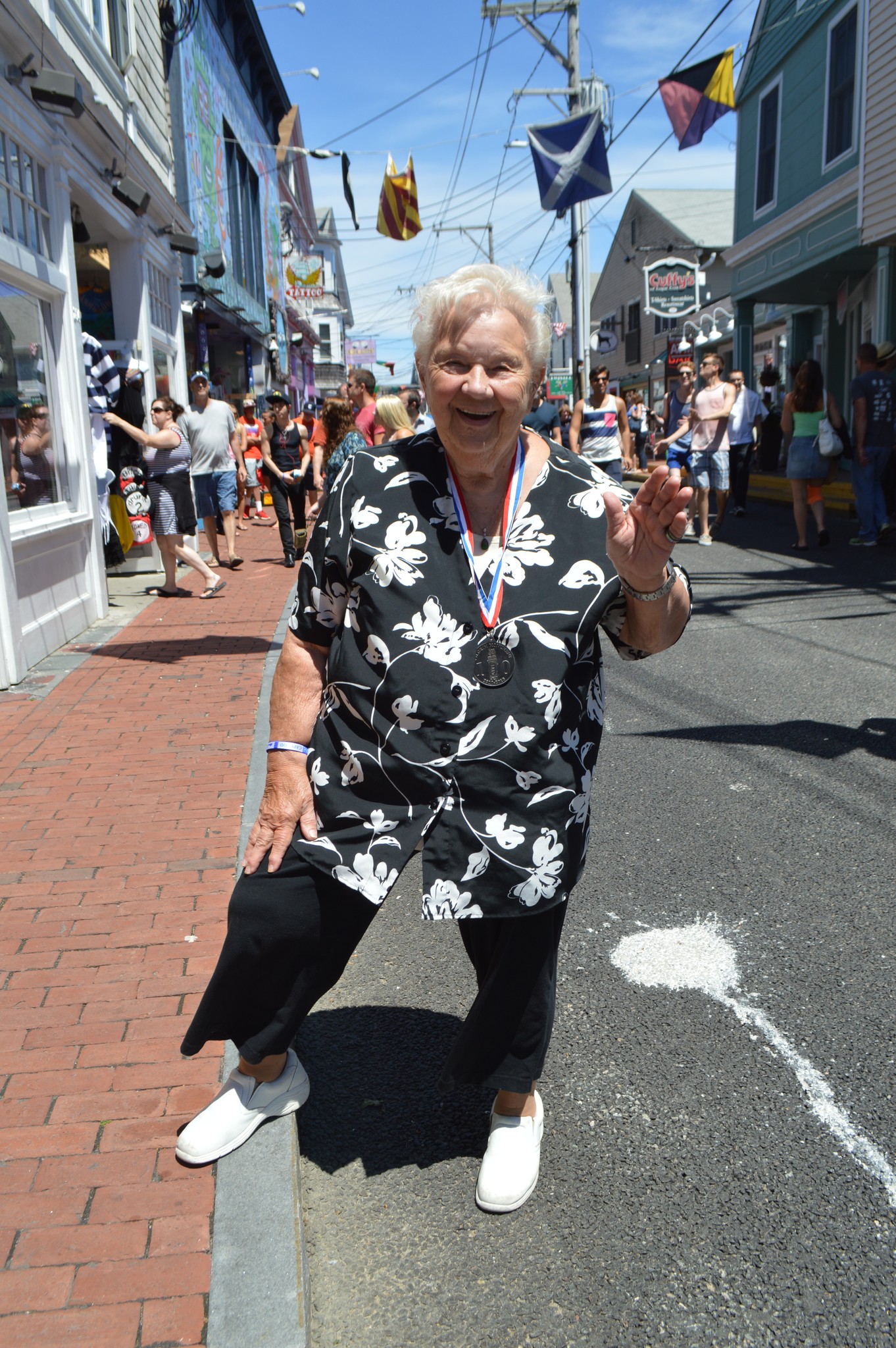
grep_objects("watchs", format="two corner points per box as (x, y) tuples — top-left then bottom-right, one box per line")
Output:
(617, 556), (675, 604)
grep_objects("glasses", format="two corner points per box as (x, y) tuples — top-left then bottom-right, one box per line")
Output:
(679, 371), (692, 375)
(151, 406), (166, 412)
(703, 361), (712, 367)
(36, 413), (49, 420)
(593, 377), (608, 381)
(347, 382), (355, 388)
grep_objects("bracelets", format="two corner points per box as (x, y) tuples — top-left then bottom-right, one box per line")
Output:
(632, 410), (635, 412)
(281, 473), (286, 482)
(266, 741), (309, 754)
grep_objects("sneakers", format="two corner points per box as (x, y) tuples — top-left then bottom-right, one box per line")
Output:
(243, 505), (250, 519)
(175, 1047), (311, 1165)
(476, 1088), (544, 1213)
(254, 510), (270, 519)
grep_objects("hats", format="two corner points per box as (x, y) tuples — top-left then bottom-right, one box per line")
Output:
(242, 398), (255, 408)
(190, 370), (209, 382)
(302, 402), (316, 414)
(266, 390), (291, 406)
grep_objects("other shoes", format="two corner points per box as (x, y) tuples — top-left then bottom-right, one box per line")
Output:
(237, 523), (247, 530)
(296, 550), (304, 559)
(684, 506), (891, 551)
(285, 554), (294, 567)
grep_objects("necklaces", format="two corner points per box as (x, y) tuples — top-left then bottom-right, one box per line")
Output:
(565, 423), (567, 425)
(448, 465), (514, 551)
(275, 420), (292, 440)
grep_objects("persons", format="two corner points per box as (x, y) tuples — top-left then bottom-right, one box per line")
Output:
(176, 369), (436, 568)
(7, 402), (57, 508)
(102, 395), (227, 599)
(780, 359), (843, 551)
(849, 341), (896, 546)
(522, 353), (786, 546)
(174, 263), (695, 1216)
(876, 341), (896, 375)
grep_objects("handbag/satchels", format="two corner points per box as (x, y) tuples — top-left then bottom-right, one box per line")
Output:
(628, 417), (643, 433)
(812, 387), (844, 457)
(294, 517), (314, 549)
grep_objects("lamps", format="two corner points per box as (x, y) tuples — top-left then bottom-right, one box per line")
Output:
(254, 0), (306, 15)
(278, 67), (319, 80)
(99, 156), (151, 217)
(198, 252), (226, 280)
(203, 287), (224, 296)
(290, 332), (303, 348)
(245, 320), (262, 326)
(5, 51), (85, 118)
(225, 306), (246, 313)
(157, 223), (199, 255)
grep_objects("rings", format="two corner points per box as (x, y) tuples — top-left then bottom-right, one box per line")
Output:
(665, 531), (680, 544)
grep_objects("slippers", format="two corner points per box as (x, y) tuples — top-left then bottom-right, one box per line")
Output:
(229, 554), (243, 568)
(200, 581), (226, 598)
(149, 588), (178, 596)
(208, 557), (220, 567)
